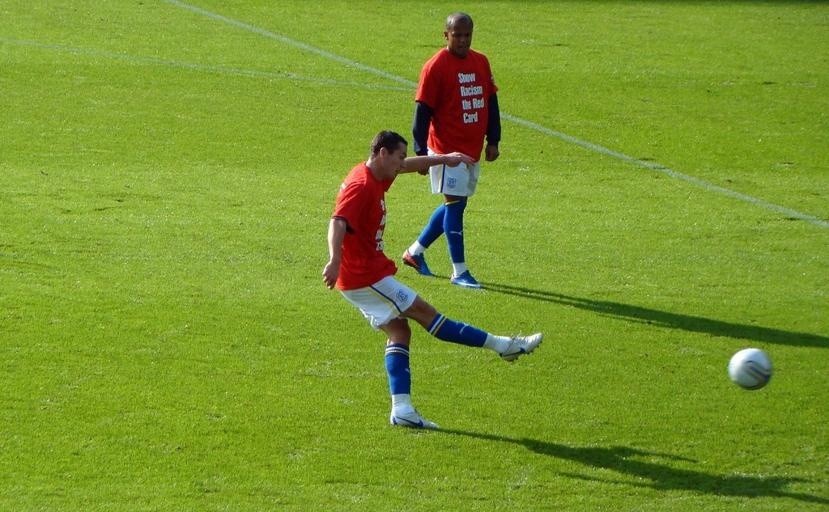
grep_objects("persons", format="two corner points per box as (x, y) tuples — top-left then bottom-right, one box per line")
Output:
(402, 12), (500, 288)
(323, 132), (543, 432)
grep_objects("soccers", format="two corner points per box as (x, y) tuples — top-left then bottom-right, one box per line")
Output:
(729, 348), (772, 390)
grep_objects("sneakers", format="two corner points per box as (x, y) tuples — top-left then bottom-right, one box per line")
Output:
(501, 333), (543, 360)
(450, 270), (481, 289)
(390, 412), (439, 429)
(402, 249), (434, 276)
